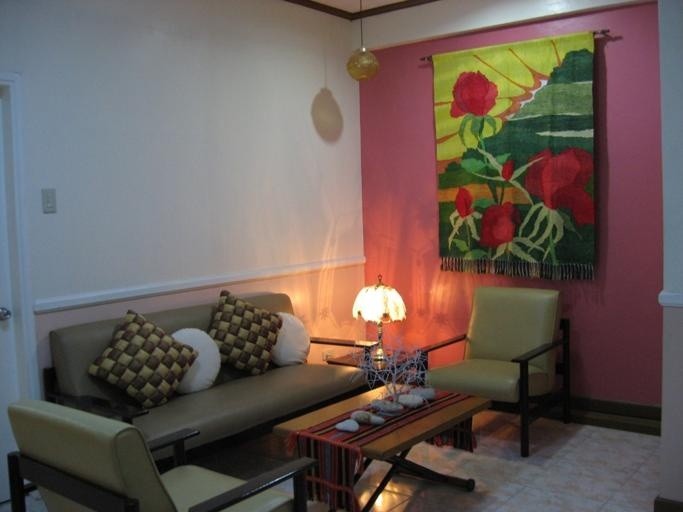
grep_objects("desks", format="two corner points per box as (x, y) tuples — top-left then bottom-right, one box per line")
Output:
(325, 349), (416, 384)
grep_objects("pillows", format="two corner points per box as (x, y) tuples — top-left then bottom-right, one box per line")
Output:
(86, 300), (194, 407)
(268, 311), (310, 367)
(167, 330), (220, 394)
(207, 291), (283, 375)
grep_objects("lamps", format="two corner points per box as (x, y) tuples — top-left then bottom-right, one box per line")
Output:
(344, 1), (379, 80)
(353, 274), (405, 360)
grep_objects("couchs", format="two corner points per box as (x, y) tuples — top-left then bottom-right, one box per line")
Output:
(5, 398), (318, 512)
(45, 292), (378, 461)
(407, 286), (572, 459)
